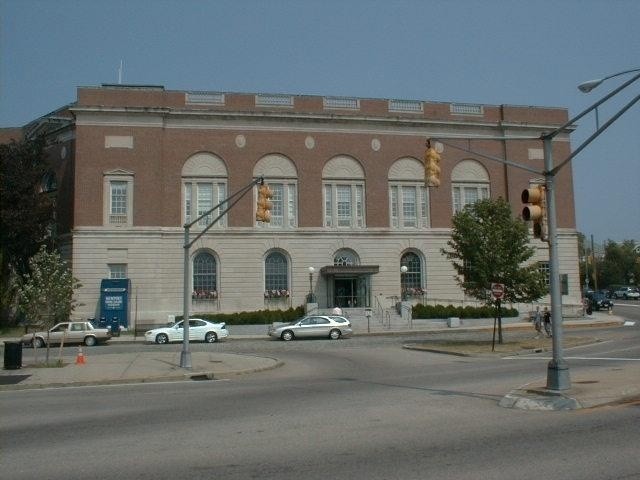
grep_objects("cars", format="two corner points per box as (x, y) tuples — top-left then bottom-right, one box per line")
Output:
(22, 318), (114, 348)
(143, 314), (233, 344)
(580, 285), (639, 309)
(268, 313), (354, 340)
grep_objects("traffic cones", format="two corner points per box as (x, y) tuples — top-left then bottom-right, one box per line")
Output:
(74, 344), (87, 365)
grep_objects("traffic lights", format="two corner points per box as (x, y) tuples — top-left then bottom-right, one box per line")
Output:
(424, 148), (443, 189)
(255, 183), (274, 223)
(521, 180), (545, 237)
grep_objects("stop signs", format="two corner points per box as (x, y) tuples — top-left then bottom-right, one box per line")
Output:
(492, 284), (503, 297)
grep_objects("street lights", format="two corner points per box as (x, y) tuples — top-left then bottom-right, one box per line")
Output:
(577, 66), (640, 93)
(306, 265), (317, 303)
(399, 265), (408, 302)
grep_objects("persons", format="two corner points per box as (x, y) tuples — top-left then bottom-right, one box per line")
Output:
(347, 289), (357, 307)
(542, 306), (552, 337)
(534, 305), (546, 340)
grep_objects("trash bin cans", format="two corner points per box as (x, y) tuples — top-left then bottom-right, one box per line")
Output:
(3, 341), (23, 370)
